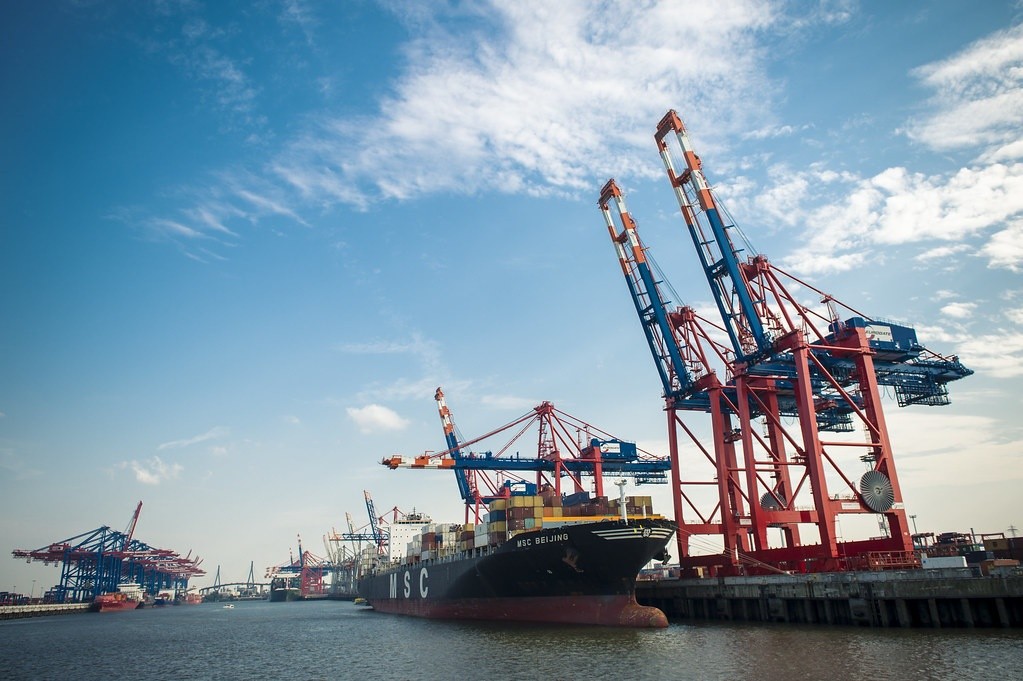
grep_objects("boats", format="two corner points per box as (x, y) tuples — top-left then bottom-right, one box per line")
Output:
(92, 590), (139, 612)
(222, 604), (235, 609)
(181, 588), (203, 604)
(149, 593), (184, 608)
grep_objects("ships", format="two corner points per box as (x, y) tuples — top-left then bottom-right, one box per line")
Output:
(360, 486), (682, 627)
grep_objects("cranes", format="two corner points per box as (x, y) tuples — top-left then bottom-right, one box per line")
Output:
(426, 388), (580, 508)
(595, 179), (869, 565)
(14, 499), (203, 602)
(654, 111), (971, 582)
(380, 403), (673, 500)
(264, 486), (392, 608)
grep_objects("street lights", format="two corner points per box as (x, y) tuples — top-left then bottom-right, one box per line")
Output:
(909, 515), (920, 533)
